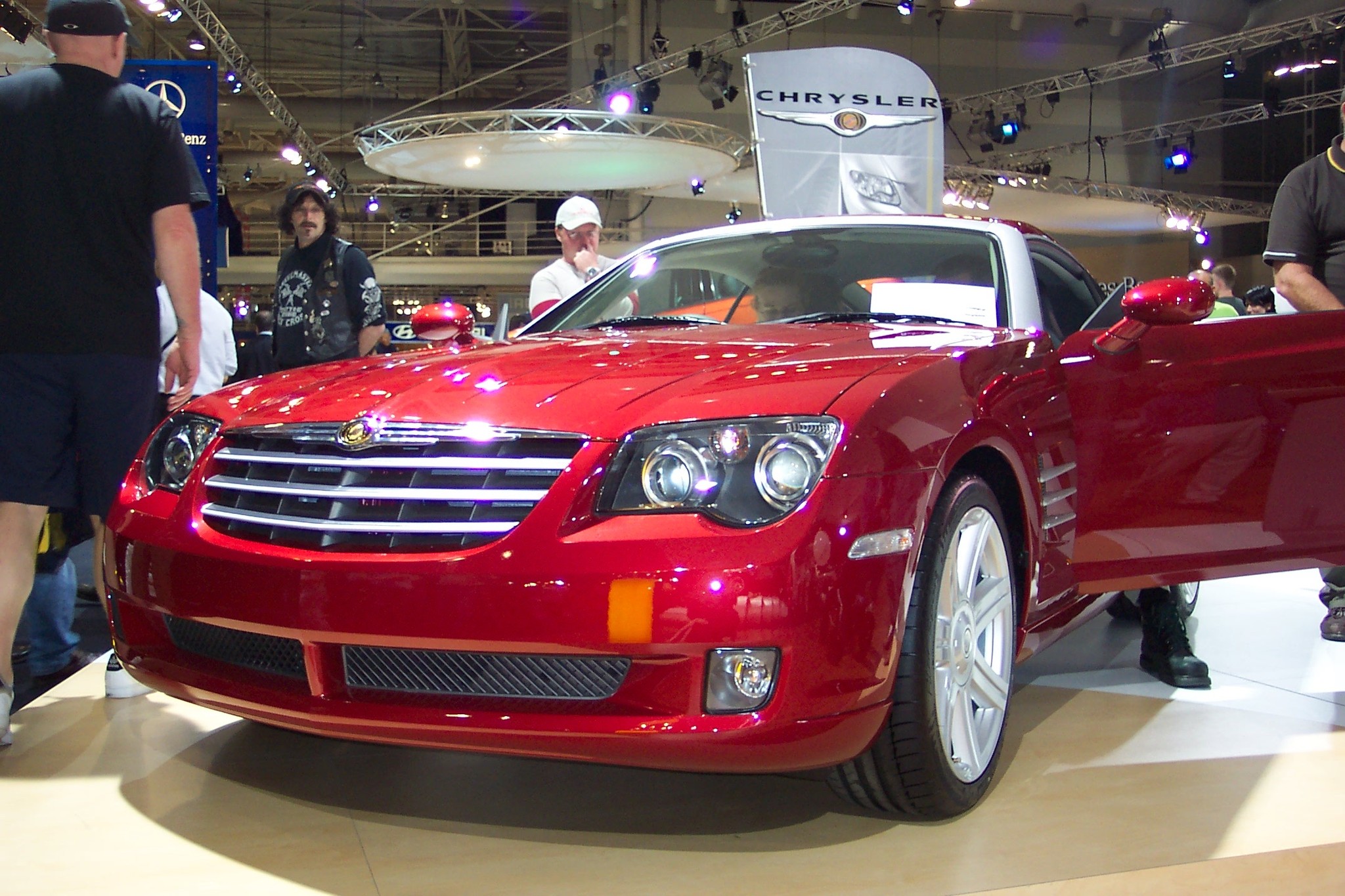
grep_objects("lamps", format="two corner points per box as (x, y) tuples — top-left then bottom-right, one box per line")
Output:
(137, 0), (1344, 239)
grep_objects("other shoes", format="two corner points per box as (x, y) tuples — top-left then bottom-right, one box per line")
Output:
(75, 583), (102, 602)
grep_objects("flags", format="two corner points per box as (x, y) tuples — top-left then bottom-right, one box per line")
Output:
(742, 47), (944, 222)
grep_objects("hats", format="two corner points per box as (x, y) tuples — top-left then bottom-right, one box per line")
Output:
(286, 181), (329, 210)
(42, 0), (132, 36)
(556, 195), (603, 232)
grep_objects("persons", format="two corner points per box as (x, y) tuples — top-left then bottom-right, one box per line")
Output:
(930, 253), (1210, 688)
(528, 196), (638, 329)
(268, 181), (386, 373)
(0, 0), (200, 748)
(1186, 262), (1277, 320)
(154, 261), (238, 422)
(754, 267), (813, 322)
(376, 329), (399, 355)
(508, 313), (533, 332)
(1262, 81), (1345, 642)
(242, 310), (274, 382)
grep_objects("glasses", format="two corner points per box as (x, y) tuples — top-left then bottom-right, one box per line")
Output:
(557, 225), (599, 242)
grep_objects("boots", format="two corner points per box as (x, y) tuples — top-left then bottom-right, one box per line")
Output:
(1140, 593), (1212, 688)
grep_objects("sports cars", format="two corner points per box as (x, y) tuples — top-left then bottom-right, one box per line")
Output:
(97, 214), (1345, 822)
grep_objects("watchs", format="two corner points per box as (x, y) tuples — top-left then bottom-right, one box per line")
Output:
(584, 267), (600, 283)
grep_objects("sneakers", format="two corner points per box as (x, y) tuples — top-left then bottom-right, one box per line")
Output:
(1320, 606), (1345, 642)
(0, 691), (15, 746)
(104, 653), (153, 699)
